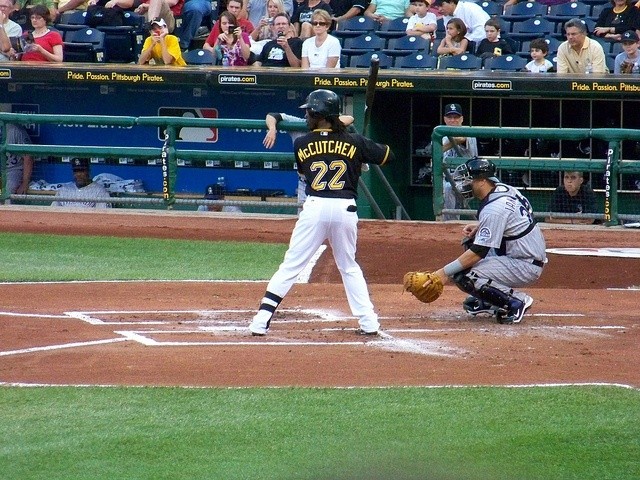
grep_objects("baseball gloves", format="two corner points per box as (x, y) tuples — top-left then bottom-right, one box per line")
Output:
(403, 270), (443, 302)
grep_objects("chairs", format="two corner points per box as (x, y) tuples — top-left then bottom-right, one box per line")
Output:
(328, 0), (624, 73)
(180, 48), (216, 65)
(46, 10), (147, 64)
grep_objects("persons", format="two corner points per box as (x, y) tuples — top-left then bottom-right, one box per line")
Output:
(0, 122), (33, 200)
(50, 157), (113, 209)
(431, 104), (478, 222)
(593, 0), (640, 42)
(20, 4), (64, 61)
(431, 0), (501, 52)
(364, 0), (415, 25)
(251, 13), (301, 68)
(212, 11), (250, 66)
(177, 0), (212, 52)
(557, 18), (610, 74)
(329, 1), (366, 30)
(436, 19), (470, 56)
(86, 1), (141, 26)
(421, 157), (549, 324)
(262, 112), (354, 218)
(54, 0), (88, 26)
(613, 30), (640, 74)
(135, 0), (184, 33)
(406, 0), (444, 19)
(502, 0), (577, 8)
(196, 184), (241, 214)
(137, 17), (187, 66)
(291, 0), (333, 38)
(519, 38), (555, 72)
(250, 0), (297, 41)
(237, 0), (294, 30)
(405, 0), (437, 41)
(545, 171), (598, 225)
(301, 10), (341, 70)
(202, 0), (253, 52)
(249, 88), (395, 335)
(0, 1), (22, 63)
(11, 0), (58, 23)
(475, 17), (512, 66)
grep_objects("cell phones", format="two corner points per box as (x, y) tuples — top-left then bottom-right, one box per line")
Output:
(276, 30), (284, 38)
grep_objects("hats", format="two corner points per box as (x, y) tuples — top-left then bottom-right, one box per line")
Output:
(621, 30), (639, 42)
(148, 17), (167, 30)
(430, 1), (443, 8)
(444, 104), (462, 116)
(204, 183), (225, 200)
(72, 157), (91, 170)
(410, 0), (432, 5)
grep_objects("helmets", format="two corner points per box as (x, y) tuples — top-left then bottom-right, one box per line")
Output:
(452, 158), (500, 199)
(299, 88), (339, 118)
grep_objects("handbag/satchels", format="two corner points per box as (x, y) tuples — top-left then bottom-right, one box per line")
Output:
(85, 6), (123, 26)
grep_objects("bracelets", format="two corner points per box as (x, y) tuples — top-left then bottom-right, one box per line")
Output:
(443, 259), (464, 278)
(0, 24), (4, 28)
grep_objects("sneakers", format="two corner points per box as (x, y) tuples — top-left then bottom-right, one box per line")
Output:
(463, 297), (508, 317)
(248, 325), (270, 335)
(498, 295), (533, 324)
(360, 329), (379, 335)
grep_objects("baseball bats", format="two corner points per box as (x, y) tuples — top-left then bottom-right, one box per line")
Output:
(362, 53), (380, 171)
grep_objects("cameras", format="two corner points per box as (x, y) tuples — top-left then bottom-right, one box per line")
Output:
(264, 16), (274, 25)
(228, 25), (238, 35)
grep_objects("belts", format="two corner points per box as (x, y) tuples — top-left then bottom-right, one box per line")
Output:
(521, 258), (547, 268)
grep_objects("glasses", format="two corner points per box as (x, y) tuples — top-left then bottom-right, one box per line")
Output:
(30, 15), (43, 20)
(565, 31), (585, 36)
(311, 22), (329, 26)
